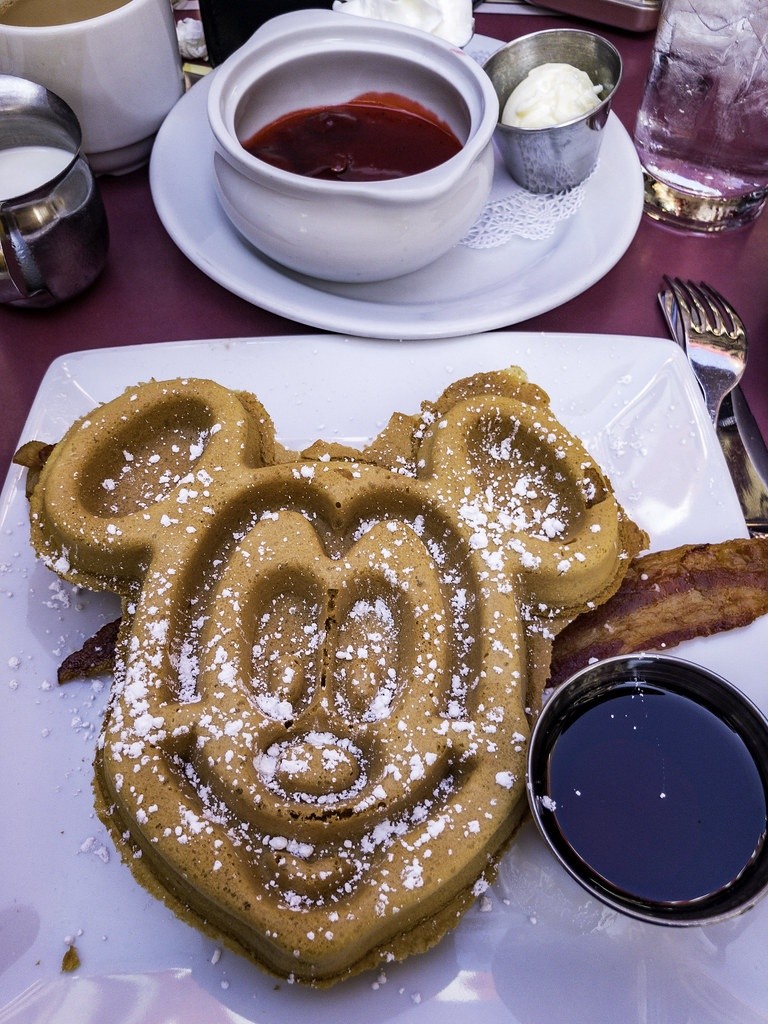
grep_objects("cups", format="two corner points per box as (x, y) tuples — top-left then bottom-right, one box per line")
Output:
(0, 73), (110, 311)
(629, 0), (768, 241)
(1, 1), (187, 177)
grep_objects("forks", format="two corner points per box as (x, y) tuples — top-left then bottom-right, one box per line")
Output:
(664, 273), (750, 431)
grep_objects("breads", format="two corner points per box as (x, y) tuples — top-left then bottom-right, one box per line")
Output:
(26, 366), (626, 990)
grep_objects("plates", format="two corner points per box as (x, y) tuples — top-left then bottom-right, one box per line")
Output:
(0, 335), (768, 1024)
(148, 29), (645, 341)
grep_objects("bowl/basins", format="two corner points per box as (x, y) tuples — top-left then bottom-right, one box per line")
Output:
(481, 28), (623, 195)
(524, 651), (768, 931)
(205, 9), (499, 283)
